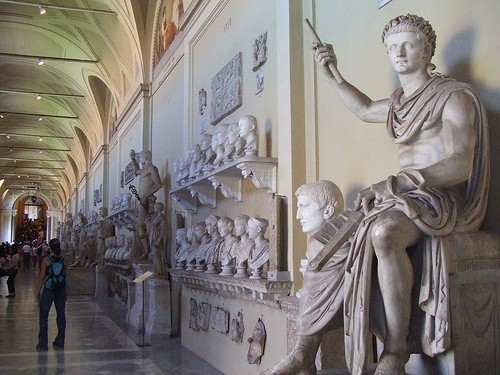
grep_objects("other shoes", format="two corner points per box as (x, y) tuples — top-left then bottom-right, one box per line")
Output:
(6, 293), (16, 298)
(36, 344), (48, 351)
(53, 342), (64, 349)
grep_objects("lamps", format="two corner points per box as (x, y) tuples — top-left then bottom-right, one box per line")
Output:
(39, 6), (46, 14)
(38, 58), (44, 66)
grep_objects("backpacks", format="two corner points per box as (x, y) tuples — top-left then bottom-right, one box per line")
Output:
(36, 246), (43, 256)
(44, 255), (65, 290)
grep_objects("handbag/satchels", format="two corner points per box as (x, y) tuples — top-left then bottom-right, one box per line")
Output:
(5, 268), (13, 276)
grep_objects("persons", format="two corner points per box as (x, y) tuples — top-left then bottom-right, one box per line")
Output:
(36, 238), (67, 351)
(56, 116), (345, 299)
(0, 217), (49, 297)
(260, 14), (492, 375)
